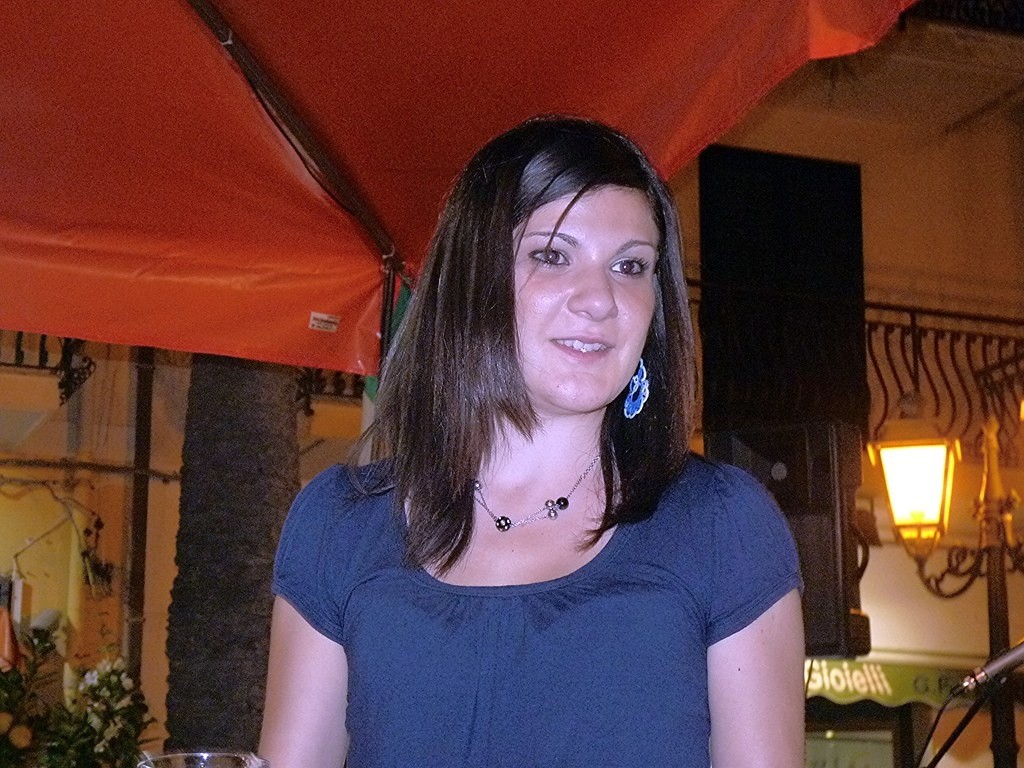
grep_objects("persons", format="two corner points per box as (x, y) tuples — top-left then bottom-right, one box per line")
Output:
(258, 114), (805, 768)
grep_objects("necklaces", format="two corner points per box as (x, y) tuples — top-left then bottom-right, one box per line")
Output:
(472, 450), (600, 530)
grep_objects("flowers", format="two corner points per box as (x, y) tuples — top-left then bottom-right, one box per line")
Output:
(0, 609), (162, 768)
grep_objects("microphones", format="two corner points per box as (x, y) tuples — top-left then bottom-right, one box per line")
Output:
(952, 640), (1024, 699)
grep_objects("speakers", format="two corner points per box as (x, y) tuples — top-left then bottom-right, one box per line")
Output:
(702, 418), (872, 661)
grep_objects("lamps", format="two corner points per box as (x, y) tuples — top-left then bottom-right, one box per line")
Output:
(878, 390), (981, 600)
